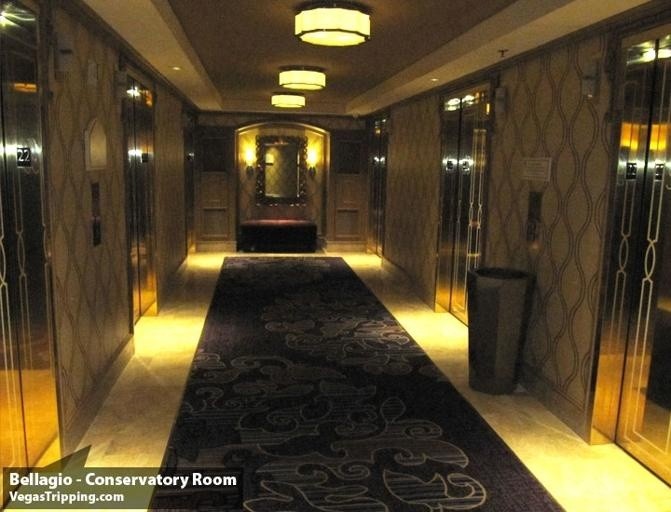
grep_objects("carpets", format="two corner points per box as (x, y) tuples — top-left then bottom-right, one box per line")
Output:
(150, 255), (566, 509)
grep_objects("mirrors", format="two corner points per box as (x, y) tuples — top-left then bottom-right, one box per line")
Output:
(255, 134), (308, 207)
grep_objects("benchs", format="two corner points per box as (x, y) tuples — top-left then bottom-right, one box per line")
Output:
(242, 219), (318, 254)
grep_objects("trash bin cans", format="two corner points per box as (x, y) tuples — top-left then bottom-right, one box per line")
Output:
(467, 266), (535, 395)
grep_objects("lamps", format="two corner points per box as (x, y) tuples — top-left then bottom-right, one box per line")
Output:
(309, 147), (318, 182)
(291, 1), (371, 49)
(270, 92), (307, 109)
(245, 147), (254, 179)
(277, 63), (328, 91)
